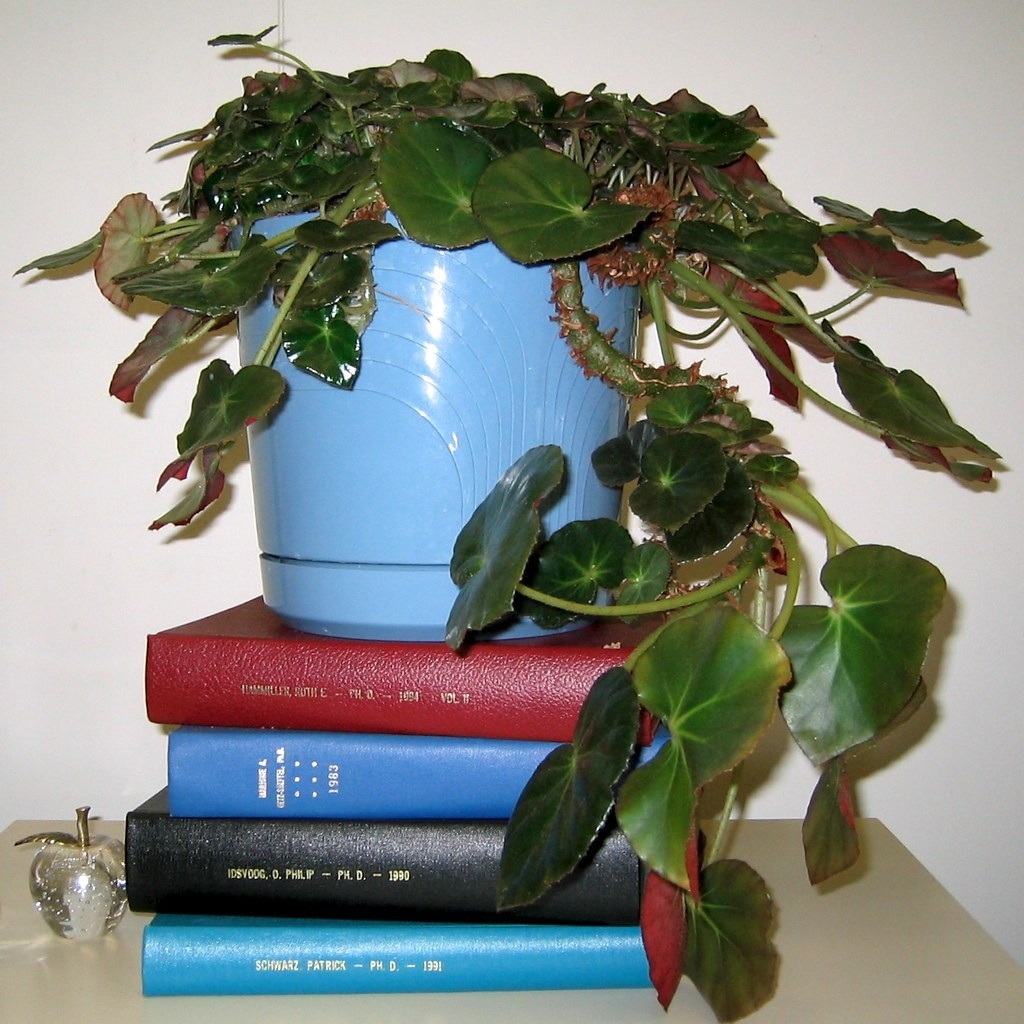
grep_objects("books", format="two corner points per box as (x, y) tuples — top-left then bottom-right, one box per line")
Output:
(124, 595), (673, 997)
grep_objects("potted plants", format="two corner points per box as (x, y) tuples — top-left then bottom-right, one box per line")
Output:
(13, 26), (1002, 1023)
